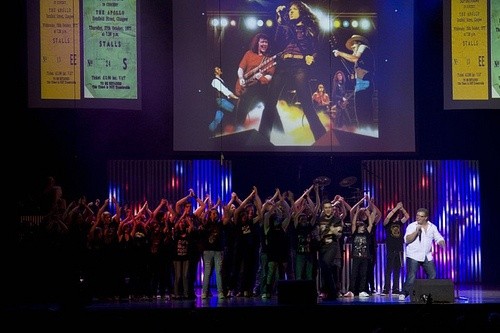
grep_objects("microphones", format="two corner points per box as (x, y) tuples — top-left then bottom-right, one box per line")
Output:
(418, 222), (422, 240)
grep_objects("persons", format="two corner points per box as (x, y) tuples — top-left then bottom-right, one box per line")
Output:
(18, 175), (382, 300)
(212, 0), (376, 142)
(403, 207), (448, 298)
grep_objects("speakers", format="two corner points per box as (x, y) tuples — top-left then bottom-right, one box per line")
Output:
(410, 279), (455, 302)
(278, 280), (318, 305)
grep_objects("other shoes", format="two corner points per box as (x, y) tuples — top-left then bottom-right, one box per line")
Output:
(218, 293), (224, 299)
(344, 292), (354, 297)
(359, 291), (369, 297)
(202, 294), (207, 299)
(399, 294), (405, 300)
(93, 294), (189, 301)
(227, 291), (272, 300)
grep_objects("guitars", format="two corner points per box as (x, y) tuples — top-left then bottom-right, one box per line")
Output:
(337, 92), (353, 110)
(235, 52), (282, 96)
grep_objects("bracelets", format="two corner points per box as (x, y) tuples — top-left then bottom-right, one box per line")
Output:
(391, 211), (394, 214)
(383, 201), (410, 297)
(404, 212), (407, 214)
